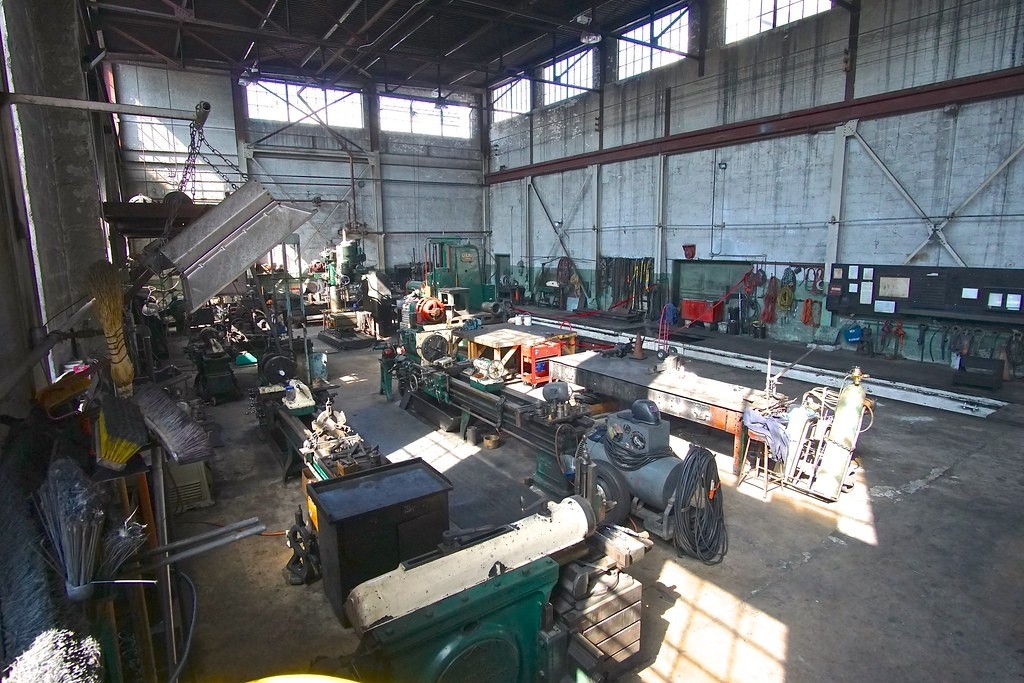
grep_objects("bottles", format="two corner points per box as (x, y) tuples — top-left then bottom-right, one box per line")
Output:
(285, 386), (294, 401)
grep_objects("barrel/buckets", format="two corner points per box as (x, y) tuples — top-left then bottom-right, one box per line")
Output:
(523, 312), (531, 326)
(309, 353), (329, 380)
(515, 315), (522, 325)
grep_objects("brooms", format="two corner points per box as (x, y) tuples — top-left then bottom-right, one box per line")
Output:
(0, 258), (217, 683)
